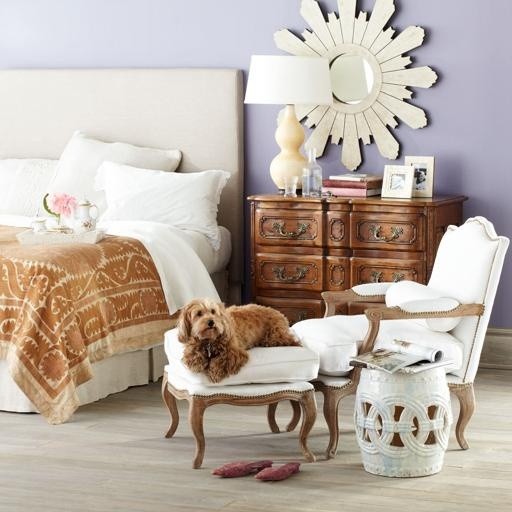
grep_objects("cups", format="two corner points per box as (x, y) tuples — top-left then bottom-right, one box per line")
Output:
(32, 217), (47, 235)
(282, 177), (298, 199)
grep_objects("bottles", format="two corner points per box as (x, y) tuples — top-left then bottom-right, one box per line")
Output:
(301, 147), (322, 197)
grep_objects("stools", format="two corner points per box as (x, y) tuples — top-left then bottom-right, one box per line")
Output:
(353, 367), (454, 478)
(160, 325), (320, 472)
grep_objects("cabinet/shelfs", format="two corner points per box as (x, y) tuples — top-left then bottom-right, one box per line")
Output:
(250, 191), (468, 327)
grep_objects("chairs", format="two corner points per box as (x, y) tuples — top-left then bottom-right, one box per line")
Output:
(292, 216), (510, 461)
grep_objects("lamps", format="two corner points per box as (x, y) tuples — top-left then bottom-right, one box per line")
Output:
(243, 54), (333, 192)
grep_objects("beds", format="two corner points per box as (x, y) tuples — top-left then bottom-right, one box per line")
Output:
(1, 67), (248, 424)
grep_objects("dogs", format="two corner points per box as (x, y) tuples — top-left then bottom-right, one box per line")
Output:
(175, 298), (303, 384)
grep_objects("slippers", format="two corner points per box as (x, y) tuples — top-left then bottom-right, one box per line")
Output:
(211, 460), (300, 480)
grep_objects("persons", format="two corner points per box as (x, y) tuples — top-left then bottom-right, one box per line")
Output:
(416, 171), (426, 190)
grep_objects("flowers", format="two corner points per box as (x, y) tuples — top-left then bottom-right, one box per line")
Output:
(45, 191), (82, 215)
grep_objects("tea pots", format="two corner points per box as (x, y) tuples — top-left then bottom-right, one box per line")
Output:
(63, 198), (100, 234)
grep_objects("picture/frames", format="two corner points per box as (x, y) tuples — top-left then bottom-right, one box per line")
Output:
(406, 156), (436, 199)
(381, 165), (415, 197)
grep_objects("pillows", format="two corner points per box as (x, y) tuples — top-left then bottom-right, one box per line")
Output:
(40, 133), (182, 221)
(2, 158), (62, 217)
(91, 161), (230, 252)
(383, 280), (463, 331)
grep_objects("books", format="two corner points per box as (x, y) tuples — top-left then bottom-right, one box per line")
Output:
(349, 339), (454, 375)
(321, 174), (382, 198)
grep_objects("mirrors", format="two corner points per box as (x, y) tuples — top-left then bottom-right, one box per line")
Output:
(276, 0), (439, 170)
